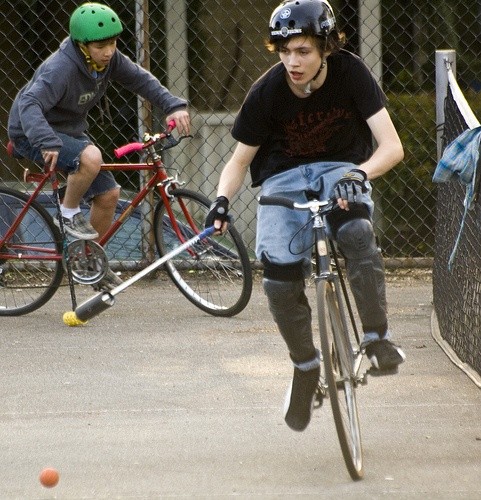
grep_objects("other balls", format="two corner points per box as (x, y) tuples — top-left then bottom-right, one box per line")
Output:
(40, 468), (58, 487)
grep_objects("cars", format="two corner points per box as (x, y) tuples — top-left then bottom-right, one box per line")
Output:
(1, 188), (238, 261)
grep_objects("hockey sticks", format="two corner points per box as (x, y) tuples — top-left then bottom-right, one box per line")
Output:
(75, 214), (236, 323)
(45, 160), (87, 326)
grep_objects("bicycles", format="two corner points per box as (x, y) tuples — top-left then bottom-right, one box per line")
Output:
(0, 118), (254, 317)
(256, 182), (400, 483)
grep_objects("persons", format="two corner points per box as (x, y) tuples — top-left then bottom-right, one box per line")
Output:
(6, 2), (190, 295)
(203, 0), (403, 431)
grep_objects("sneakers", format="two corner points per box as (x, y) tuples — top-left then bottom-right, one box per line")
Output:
(366, 338), (405, 369)
(53, 212), (98, 240)
(282, 366), (322, 433)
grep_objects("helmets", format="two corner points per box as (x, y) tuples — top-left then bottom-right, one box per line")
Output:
(267, 0), (337, 42)
(70, 2), (123, 42)
